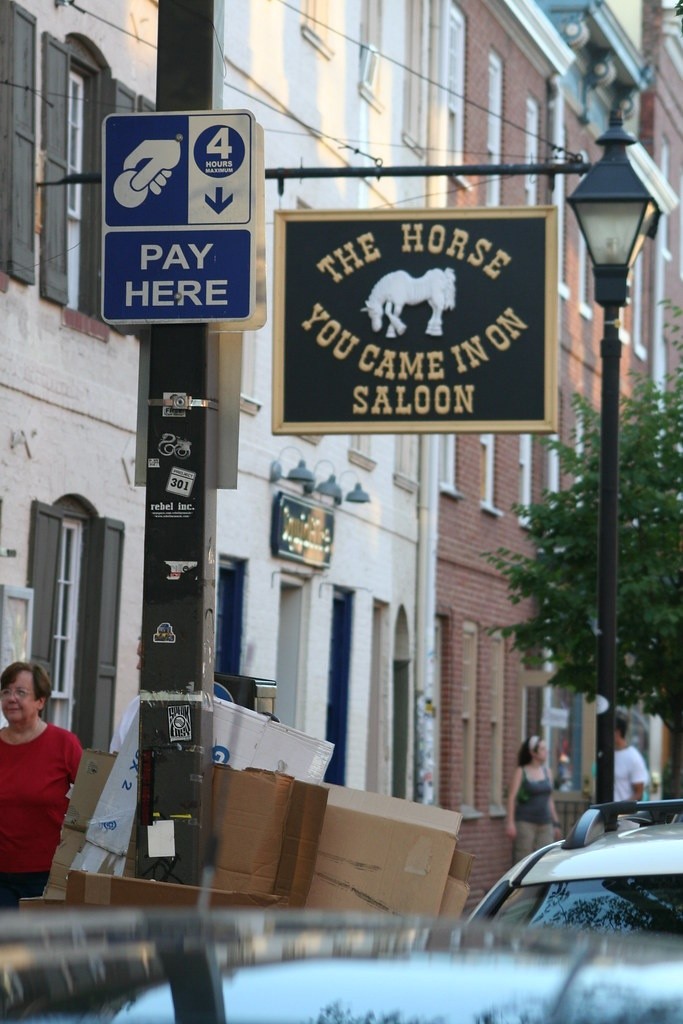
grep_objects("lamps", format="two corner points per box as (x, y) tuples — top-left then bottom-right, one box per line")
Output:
(269, 457), (371, 506)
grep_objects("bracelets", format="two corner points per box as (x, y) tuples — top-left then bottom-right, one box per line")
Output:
(553, 823), (560, 827)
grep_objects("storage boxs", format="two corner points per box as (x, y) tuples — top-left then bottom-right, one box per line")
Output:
(18, 694), (476, 915)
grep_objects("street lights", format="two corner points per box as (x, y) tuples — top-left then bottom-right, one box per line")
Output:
(560, 104), (661, 830)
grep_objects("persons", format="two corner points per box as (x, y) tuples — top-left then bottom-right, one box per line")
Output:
(0, 662), (82, 907)
(506, 736), (561, 864)
(614, 711), (648, 801)
(111, 636), (142, 750)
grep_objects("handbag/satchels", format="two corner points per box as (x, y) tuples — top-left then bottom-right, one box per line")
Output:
(515, 767), (531, 802)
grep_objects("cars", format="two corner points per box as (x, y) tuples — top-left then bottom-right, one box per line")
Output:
(0, 911), (683, 1024)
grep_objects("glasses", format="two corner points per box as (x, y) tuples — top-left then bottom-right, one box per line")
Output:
(0, 687), (35, 699)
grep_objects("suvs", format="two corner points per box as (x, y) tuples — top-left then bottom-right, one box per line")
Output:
(464, 796), (682, 950)
